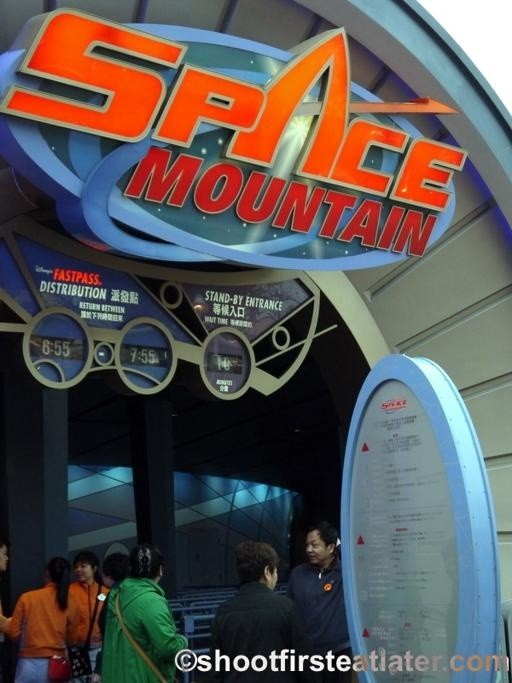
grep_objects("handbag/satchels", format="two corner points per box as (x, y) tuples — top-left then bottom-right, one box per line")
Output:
(47, 647), (92, 683)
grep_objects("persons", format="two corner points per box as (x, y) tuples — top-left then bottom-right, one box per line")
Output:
(96, 541), (191, 682)
(90, 550), (133, 682)
(1, 540), (22, 683)
(281, 520), (352, 681)
(62, 549), (111, 682)
(206, 537), (312, 682)
(1, 554), (83, 682)
